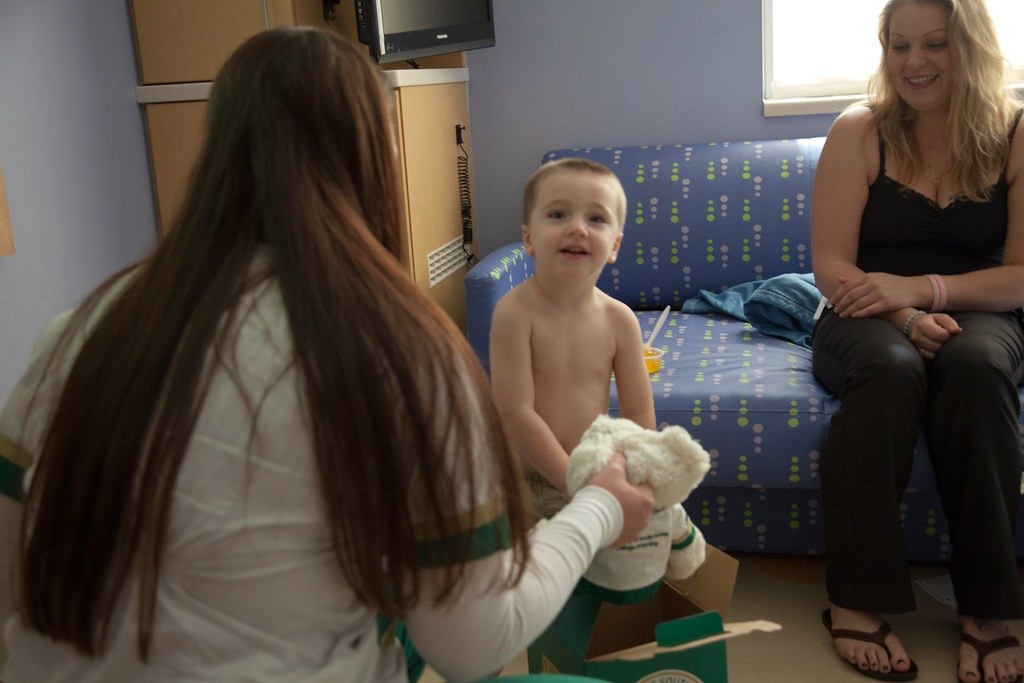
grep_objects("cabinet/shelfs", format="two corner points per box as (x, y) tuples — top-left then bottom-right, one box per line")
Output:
(126, 0), (475, 334)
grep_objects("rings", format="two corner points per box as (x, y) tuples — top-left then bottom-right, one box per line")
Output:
(919, 348), (926, 358)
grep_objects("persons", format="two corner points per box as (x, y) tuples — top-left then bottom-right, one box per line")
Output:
(0, 28), (657, 683)
(811, 0), (1024, 683)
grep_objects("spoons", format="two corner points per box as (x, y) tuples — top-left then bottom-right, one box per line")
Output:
(643, 306), (671, 351)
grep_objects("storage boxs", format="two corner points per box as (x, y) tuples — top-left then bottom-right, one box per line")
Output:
(528, 550), (783, 683)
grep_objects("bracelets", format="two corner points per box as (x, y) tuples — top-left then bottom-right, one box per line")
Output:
(901, 311), (926, 335)
(925, 274), (948, 313)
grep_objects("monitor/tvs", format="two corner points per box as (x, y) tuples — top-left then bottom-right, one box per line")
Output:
(355, 0), (496, 65)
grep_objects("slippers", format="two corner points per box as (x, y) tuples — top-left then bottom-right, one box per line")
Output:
(956, 612), (1024, 683)
(822, 607), (918, 681)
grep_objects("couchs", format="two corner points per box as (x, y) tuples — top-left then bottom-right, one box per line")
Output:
(464, 138), (950, 563)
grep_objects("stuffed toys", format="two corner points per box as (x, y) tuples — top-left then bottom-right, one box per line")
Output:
(565, 414), (711, 606)
